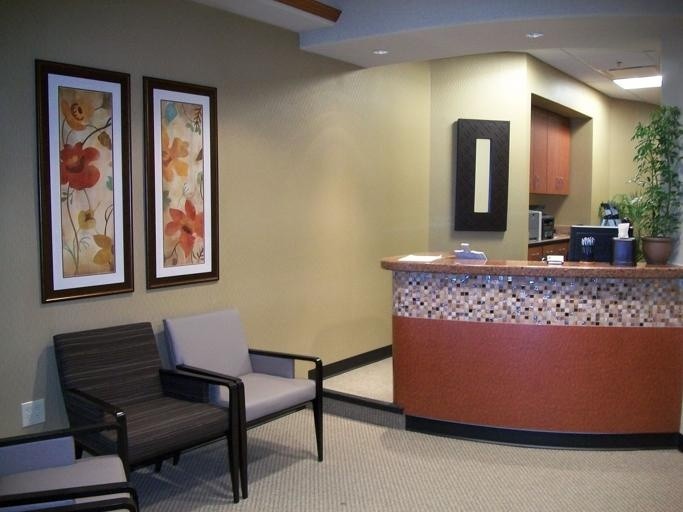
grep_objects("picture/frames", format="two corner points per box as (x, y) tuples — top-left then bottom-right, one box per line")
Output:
(140, 74), (220, 291)
(34, 60), (134, 303)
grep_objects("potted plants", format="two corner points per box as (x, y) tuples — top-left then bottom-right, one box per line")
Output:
(625, 103), (683, 265)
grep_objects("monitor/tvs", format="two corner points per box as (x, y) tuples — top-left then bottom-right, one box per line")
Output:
(568, 225), (633, 261)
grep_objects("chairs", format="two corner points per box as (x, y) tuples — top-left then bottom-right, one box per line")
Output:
(52, 321), (239, 502)
(163, 308), (323, 498)
(0, 421), (139, 512)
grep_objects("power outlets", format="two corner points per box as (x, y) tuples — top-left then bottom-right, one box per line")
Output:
(20, 398), (46, 428)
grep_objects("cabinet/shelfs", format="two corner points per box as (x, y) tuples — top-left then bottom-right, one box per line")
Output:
(530, 105), (570, 196)
(528, 234), (569, 261)
